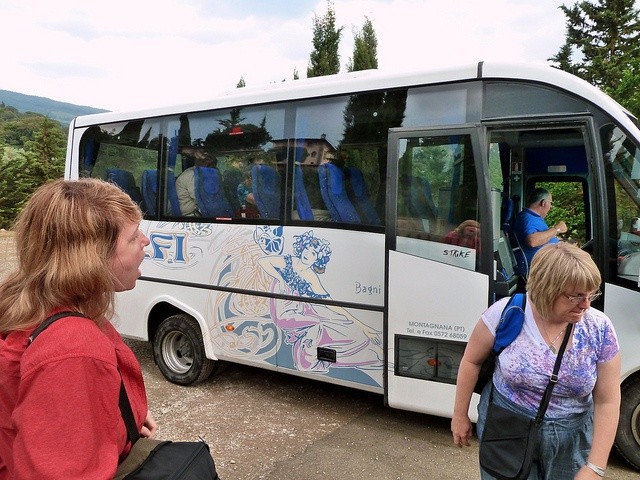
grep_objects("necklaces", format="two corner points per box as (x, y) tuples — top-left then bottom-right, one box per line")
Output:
(541, 317), (567, 354)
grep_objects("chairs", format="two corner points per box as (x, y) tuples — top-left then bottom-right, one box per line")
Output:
(105, 166), (144, 221)
(344, 164), (386, 228)
(193, 166), (238, 223)
(144, 168), (175, 218)
(317, 163), (363, 226)
(291, 162), (316, 222)
(250, 163), (285, 221)
(223, 166), (252, 220)
(418, 175), (440, 224)
(399, 174), (437, 231)
(499, 195), (540, 286)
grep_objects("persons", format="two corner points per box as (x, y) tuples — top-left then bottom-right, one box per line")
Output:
(511, 187), (567, 274)
(258, 230), (386, 375)
(442, 221), (480, 251)
(451, 244), (620, 479)
(0, 178), (157, 480)
(237, 172), (255, 211)
(176, 149), (218, 217)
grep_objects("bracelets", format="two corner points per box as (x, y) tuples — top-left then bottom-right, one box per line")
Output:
(554, 226), (560, 235)
(587, 462), (606, 478)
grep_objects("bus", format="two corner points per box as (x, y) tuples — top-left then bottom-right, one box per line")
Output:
(63, 61), (640, 472)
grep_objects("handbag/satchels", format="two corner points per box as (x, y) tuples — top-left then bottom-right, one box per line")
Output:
(479, 322), (573, 480)
(27, 311), (219, 480)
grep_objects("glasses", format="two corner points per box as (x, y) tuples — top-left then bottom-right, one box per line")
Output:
(545, 200), (552, 205)
(561, 290), (602, 304)
